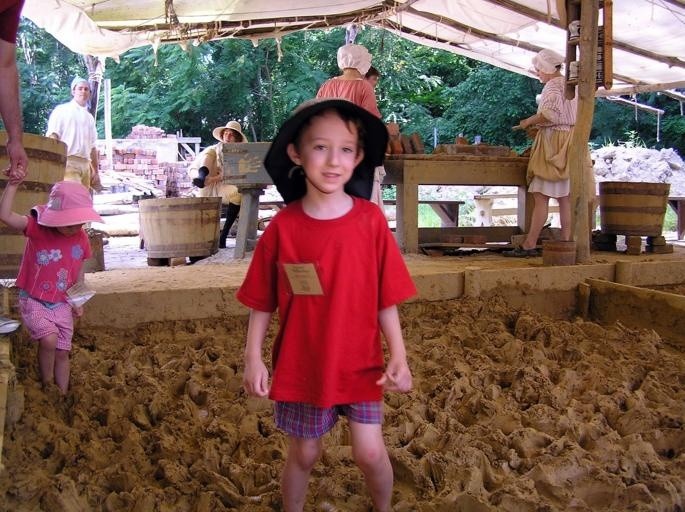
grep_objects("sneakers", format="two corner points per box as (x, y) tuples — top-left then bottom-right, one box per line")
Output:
(0, 314), (22, 335)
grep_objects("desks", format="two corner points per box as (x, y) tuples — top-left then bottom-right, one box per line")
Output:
(379, 155), (596, 253)
(219, 140), (276, 260)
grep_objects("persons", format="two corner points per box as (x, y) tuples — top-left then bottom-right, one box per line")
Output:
(365, 67), (379, 88)
(46, 78), (101, 189)
(315, 45), (386, 206)
(190, 121), (248, 248)
(0, 164), (105, 394)
(502, 48), (578, 258)
(234, 97), (419, 512)
(0, 0), (29, 332)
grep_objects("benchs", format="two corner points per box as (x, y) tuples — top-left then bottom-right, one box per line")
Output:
(471, 191), (562, 228)
(214, 199), (466, 237)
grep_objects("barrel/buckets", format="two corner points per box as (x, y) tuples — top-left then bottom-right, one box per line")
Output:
(0, 132), (66, 278)
(600, 180), (671, 237)
(541, 239), (577, 266)
(138, 197), (224, 259)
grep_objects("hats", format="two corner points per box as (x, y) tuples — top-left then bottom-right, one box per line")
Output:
(532, 48), (566, 74)
(29, 180), (106, 228)
(261, 98), (390, 200)
(335, 42), (373, 76)
(213, 120), (248, 144)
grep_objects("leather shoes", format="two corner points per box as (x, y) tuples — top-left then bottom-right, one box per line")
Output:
(502, 245), (539, 257)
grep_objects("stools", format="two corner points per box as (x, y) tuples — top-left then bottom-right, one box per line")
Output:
(667, 195), (685, 241)
